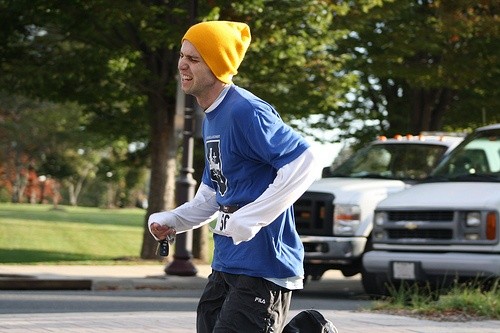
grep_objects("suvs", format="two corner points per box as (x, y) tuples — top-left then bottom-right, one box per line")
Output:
(293, 135), (467, 281)
(361, 121), (500, 296)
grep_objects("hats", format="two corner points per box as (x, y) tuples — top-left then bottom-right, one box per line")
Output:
(180, 20), (251, 84)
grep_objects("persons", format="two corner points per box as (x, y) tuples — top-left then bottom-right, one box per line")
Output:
(146, 19), (340, 333)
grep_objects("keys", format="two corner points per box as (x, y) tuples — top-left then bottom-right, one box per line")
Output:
(155, 227), (176, 256)
